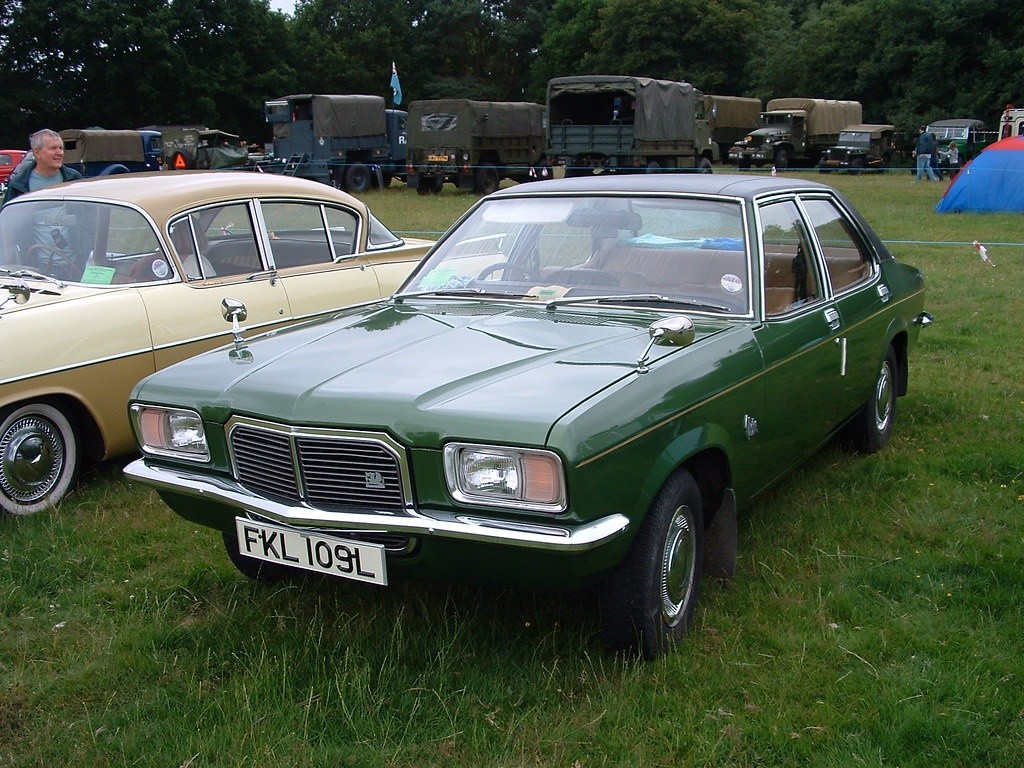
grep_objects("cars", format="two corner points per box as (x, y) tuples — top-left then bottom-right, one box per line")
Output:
(0, 173), (508, 517)
(122, 172), (936, 663)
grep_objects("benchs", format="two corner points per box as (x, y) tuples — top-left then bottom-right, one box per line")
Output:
(601, 246), (863, 290)
(207, 237), (351, 276)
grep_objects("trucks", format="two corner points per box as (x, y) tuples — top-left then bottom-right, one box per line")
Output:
(702, 94), (763, 163)
(58, 130), (164, 181)
(729, 98), (863, 171)
(141, 125), (248, 171)
(404, 98), (553, 195)
(547, 76), (720, 177)
(265, 93), (410, 191)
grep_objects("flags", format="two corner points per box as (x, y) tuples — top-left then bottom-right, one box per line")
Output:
(390, 61), (401, 104)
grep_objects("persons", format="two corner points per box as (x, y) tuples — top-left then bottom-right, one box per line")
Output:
(128, 216), (216, 281)
(938, 141), (960, 179)
(912, 125), (945, 182)
(0, 128), (95, 283)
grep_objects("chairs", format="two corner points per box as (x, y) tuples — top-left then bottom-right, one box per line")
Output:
(679, 283), (797, 314)
(535, 266), (617, 288)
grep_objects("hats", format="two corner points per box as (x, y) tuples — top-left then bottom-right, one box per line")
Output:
(947, 142), (956, 148)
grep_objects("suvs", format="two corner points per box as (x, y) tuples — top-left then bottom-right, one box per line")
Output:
(818, 124), (894, 173)
(912, 119), (986, 178)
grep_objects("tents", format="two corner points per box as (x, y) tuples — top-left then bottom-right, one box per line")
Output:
(934, 134), (1024, 213)
